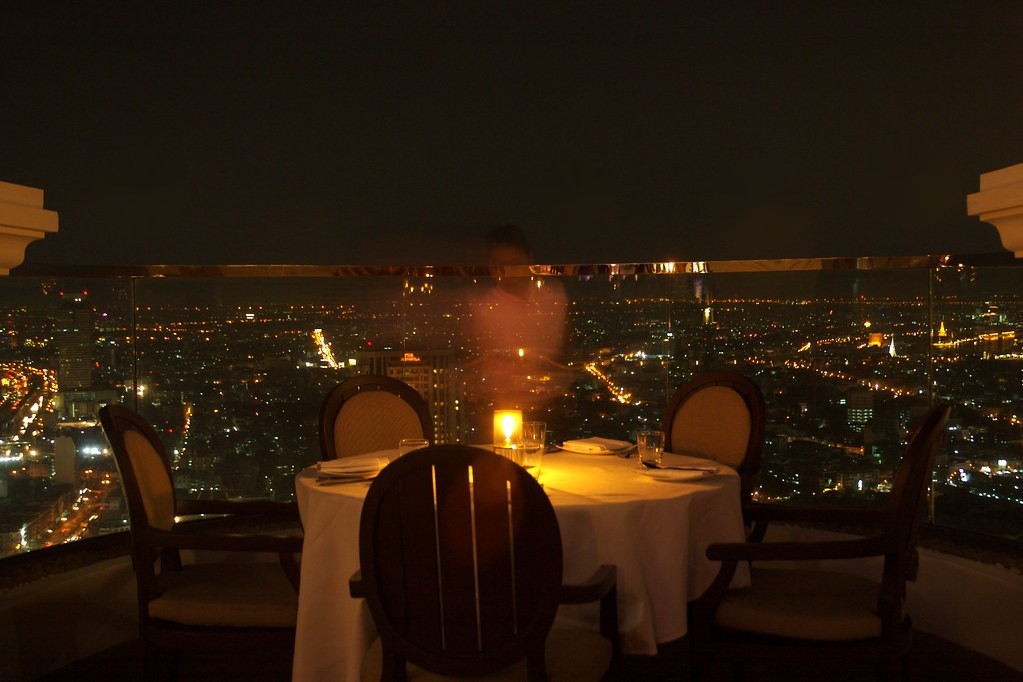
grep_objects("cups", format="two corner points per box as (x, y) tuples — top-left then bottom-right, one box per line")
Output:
(494, 409), (523, 460)
(399, 438), (428, 456)
(511, 421), (546, 478)
(637, 431), (665, 464)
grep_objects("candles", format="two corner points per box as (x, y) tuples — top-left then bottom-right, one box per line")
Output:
(494, 408), (527, 467)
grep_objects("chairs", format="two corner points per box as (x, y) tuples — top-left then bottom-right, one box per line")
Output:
(661, 372), (771, 492)
(99, 405), (304, 682)
(684, 403), (951, 682)
(319, 374), (436, 462)
(348, 444), (625, 682)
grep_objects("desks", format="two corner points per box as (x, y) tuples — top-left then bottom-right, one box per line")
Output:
(292, 443), (754, 682)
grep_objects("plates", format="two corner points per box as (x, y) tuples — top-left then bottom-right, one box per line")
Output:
(316, 476), (367, 486)
(633, 465), (719, 481)
(554, 444), (638, 454)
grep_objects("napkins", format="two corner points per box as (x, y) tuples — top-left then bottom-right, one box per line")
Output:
(636, 460), (721, 479)
(314, 457), (391, 476)
(564, 436), (632, 453)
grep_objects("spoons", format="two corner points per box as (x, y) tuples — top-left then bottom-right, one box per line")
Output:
(642, 461), (714, 472)
